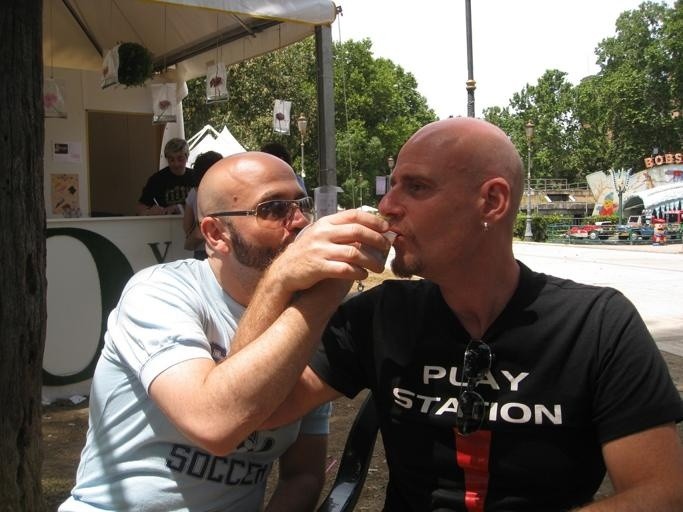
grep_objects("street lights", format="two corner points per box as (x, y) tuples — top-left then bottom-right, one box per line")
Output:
(523, 120), (535, 242)
(297, 112), (308, 183)
(388, 156), (394, 189)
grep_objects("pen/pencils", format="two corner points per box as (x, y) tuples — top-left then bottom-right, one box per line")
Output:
(153, 198), (160, 206)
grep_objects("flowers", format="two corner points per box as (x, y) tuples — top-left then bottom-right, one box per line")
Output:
(275, 112), (285, 131)
(43, 93), (62, 113)
(209, 76), (223, 98)
(155, 100), (171, 123)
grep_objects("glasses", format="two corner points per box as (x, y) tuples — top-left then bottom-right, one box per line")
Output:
(454, 338), (493, 437)
(206, 196), (316, 229)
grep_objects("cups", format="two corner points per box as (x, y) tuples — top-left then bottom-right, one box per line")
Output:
(356, 230), (398, 266)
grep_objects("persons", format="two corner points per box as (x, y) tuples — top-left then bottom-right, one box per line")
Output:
(136, 137), (196, 216)
(228, 117), (683, 512)
(57, 150), (383, 512)
(261, 144), (307, 196)
(183, 151), (224, 259)
